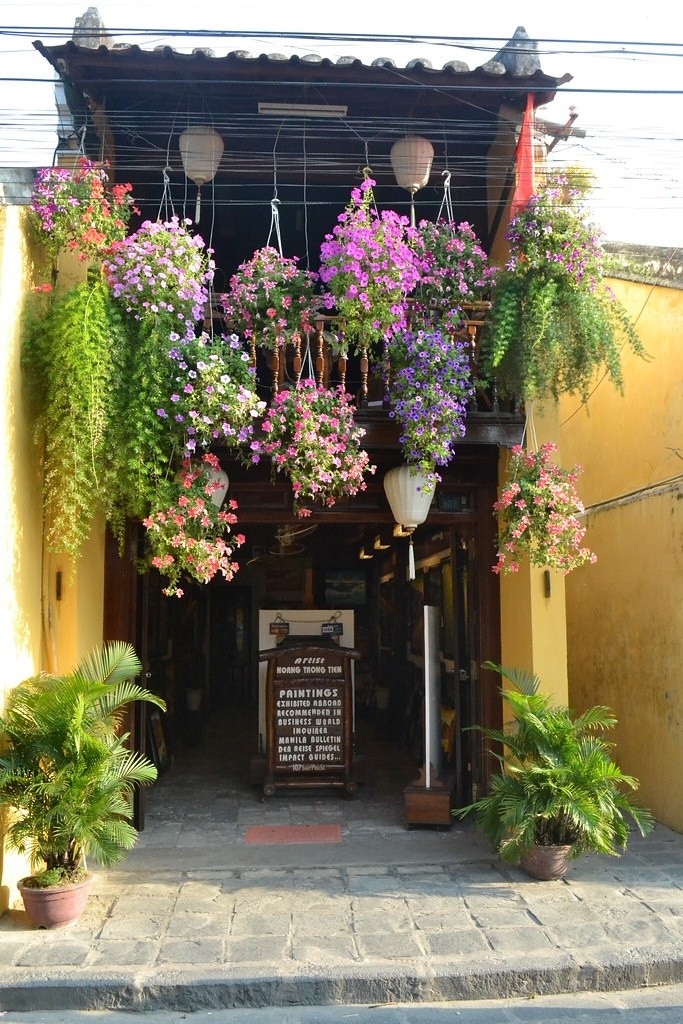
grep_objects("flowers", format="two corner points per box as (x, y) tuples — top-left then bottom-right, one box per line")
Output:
(225, 242), (333, 347)
(251, 378), (377, 519)
(101, 218), (214, 334)
(136, 469), (243, 598)
(491, 442), (597, 576)
(33, 157), (139, 266)
(319, 179), (420, 342)
(421, 216), (499, 308)
(169, 336), (266, 460)
(390, 310), (477, 500)
(508, 175), (613, 295)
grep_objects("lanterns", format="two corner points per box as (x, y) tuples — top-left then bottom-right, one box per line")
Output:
(389, 135), (435, 230)
(384, 461), (438, 581)
(175, 461), (229, 520)
(179, 125), (225, 224)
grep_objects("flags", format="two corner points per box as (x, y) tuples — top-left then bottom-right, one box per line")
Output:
(510, 93), (536, 217)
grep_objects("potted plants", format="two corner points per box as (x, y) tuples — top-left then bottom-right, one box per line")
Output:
(451, 662), (654, 882)
(0, 643), (166, 930)
